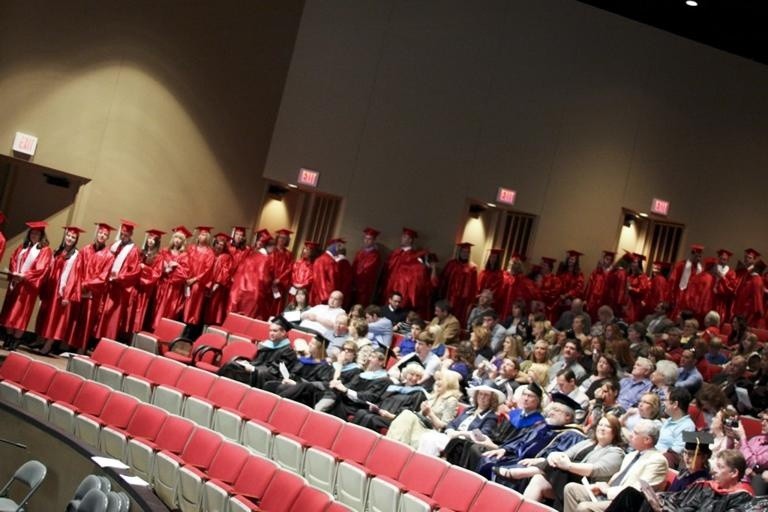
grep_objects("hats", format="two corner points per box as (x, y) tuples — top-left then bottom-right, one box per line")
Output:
(690, 245), (761, 265)
(363, 228), (379, 236)
(456, 243), (474, 249)
(374, 340), (395, 359)
(464, 380), (506, 405)
(682, 431), (714, 450)
(527, 383), (580, 410)
(603, 250), (663, 265)
(488, 248), (583, 262)
(314, 332), (329, 348)
(25, 219), (137, 232)
(398, 354), (425, 374)
(146, 226), (293, 241)
(271, 314), (294, 332)
(304, 238), (346, 246)
(403, 228), (417, 237)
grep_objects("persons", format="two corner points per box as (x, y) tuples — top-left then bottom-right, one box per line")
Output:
(1, 220), (768, 359)
(220, 291), (768, 512)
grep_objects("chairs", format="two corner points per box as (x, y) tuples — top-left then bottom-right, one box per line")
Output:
(1, 308), (768, 511)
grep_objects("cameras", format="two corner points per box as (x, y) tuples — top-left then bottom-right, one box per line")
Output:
(722, 417), (738, 427)
